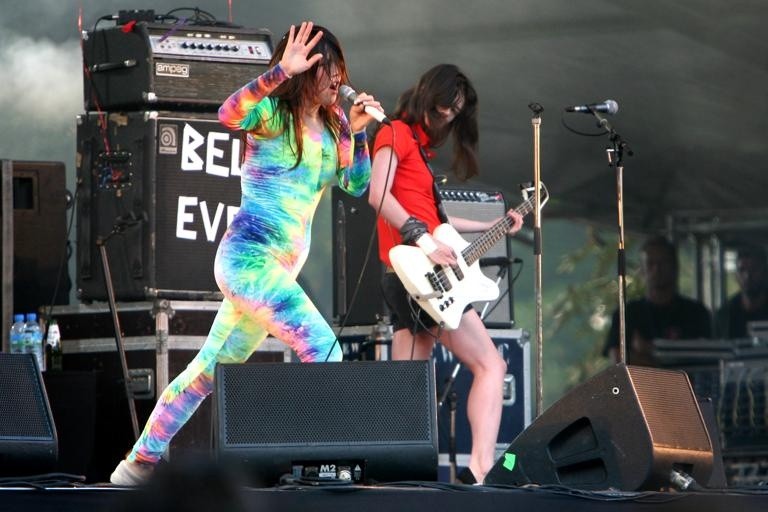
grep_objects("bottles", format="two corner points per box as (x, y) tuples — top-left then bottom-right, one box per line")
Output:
(10, 313), (63, 375)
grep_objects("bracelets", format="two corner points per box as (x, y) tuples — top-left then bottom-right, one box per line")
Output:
(398, 216), (429, 244)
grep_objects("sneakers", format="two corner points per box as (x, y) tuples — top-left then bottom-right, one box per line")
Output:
(455, 467), (477, 485)
(110, 460), (154, 487)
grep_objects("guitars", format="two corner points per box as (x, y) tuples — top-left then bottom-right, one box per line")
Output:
(389, 181), (550, 332)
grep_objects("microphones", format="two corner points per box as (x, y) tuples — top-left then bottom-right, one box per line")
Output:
(480, 256), (522, 265)
(564, 99), (619, 116)
(339, 85), (392, 125)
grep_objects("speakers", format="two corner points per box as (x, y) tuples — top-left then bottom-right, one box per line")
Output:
(214, 360), (438, 480)
(483, 363), (714, 492)
(0, 354), (69, 486)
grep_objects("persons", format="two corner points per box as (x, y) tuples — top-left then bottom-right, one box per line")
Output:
(109, 20), (385, 488)
(366, 64), (524, 485)
(602, 236), (711, 375)
(715, 241), (767, 338)
